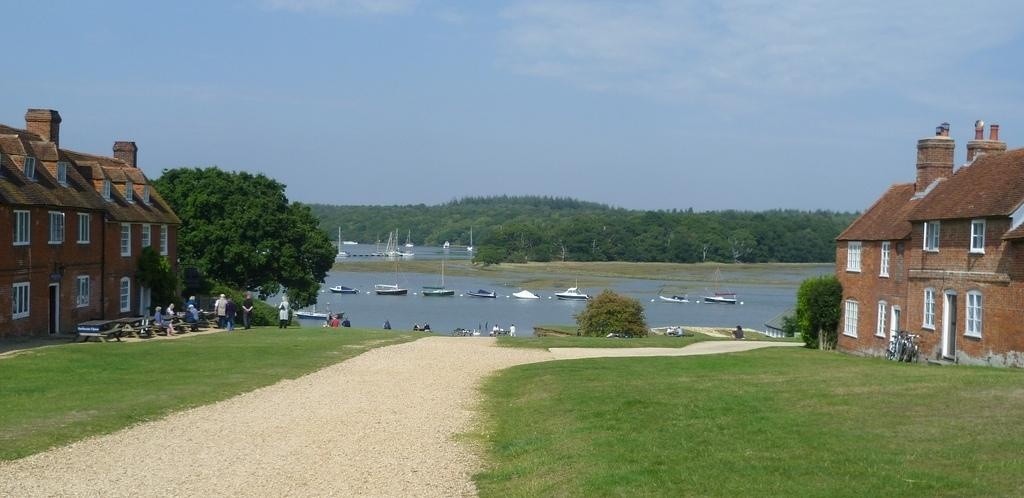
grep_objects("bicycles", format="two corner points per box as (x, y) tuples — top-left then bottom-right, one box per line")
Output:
(884, 329), (926, 363)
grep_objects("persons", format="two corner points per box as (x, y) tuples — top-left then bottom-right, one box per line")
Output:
(493, 325), (501, 337)
(731, 325), (743, 339)
(509, 324), (516, 337)
(423, 322), (429, 332)
(326, 311), (350, 328)
(154, 290), (254, 337)
(414, 323), (420, 331)
(381, 319), (391, 329)
(278, 295), (291, 329)
(666, 326), (683, 336)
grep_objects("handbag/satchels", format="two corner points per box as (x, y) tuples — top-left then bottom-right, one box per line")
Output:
(281, 304), (284, 309)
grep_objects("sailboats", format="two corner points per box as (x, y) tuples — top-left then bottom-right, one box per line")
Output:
(372, 227), (415, 257)
(466, 225), (474, 252)
(374, 256), (411, 295)
(423, 255), (455, 297)
(336, 227), (351, 257)
(703, 265), (737, 303)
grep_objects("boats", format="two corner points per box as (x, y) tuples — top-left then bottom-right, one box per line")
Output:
(329, 285), (357, 293)
(659, 296), (690, 302)
(443, 240), (450, 249)
(295, 311), (333, 321)
(556, 288), (590, 300)
(467, 289), (497, 297)
(342, 241), (358, 244)
(513, 290), (541, 299)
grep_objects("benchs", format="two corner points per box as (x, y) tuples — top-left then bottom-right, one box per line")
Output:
(76, 311), (215, 342)
(73, 328), (121, 337)
